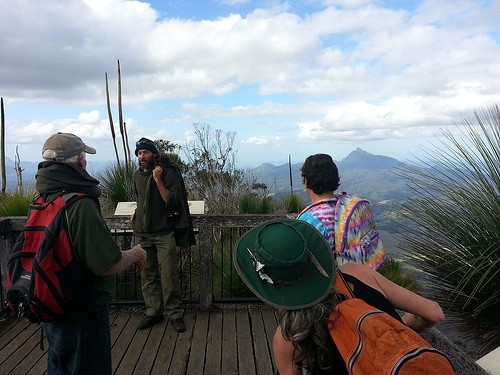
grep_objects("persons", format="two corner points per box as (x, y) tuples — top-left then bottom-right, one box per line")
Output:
(35, 134), (146, 375)
(294, 154), (387, 270)
(131, 138), (186, 332)
(231, 217), (445, 375)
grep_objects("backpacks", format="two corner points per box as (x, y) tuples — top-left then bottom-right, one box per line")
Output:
(7, 191), (101, 323)
(295, 191), (387, 270)
(324, 269), (456, 375)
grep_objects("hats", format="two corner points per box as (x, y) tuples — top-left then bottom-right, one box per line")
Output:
(43, 132), (96, 161)
(233, 219), (334, 309)
(136, 138), (157, 153)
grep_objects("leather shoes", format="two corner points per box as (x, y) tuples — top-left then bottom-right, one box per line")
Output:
(170, 314), (186, 332)
(138, 313), (162, 329)
